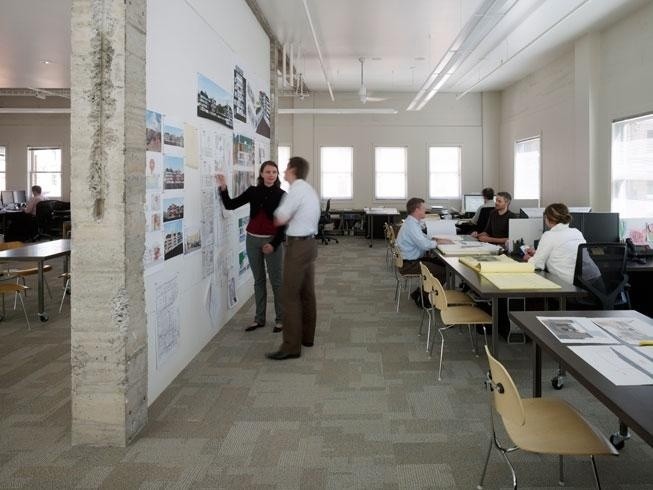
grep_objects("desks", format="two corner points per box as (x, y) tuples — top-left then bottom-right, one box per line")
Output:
(316, 200), (497, 247)
(426, 234), (588, 390)
(507, 310), (653, 451)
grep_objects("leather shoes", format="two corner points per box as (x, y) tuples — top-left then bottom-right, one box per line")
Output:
(300, 341), (314, 347)
(265, 350), (301, 360)
(410, 289), (431, 309)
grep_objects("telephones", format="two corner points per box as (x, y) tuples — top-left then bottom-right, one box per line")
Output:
(626, 237), (653, 257)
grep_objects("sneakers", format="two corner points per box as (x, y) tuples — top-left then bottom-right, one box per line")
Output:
(245, 321), (265, 331)
(273, 327), (283, 332)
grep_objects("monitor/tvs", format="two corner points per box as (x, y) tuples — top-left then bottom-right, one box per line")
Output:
(463, 193), (497, 213)
(543, 213), (620, 243)
(12, 190), (26, 205)
(520, 207), (593, 219)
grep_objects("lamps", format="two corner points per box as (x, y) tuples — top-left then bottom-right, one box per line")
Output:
(405, 0), (517, 111)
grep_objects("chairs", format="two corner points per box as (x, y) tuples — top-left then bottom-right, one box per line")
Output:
(384, 222), (424, 311)
(419, 261), (493, 380)
(0, 200), (71, 331)
(571, 241), (630, 310)
(478, 342), (620, 490)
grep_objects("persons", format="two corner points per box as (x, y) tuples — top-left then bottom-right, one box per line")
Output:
(523, 203), (606, 311)
(469, 187), (498, 226)
(393, 198), (455, 310)
(20, 185), (45, 242)
(218, 160), (290, 333)
(265, 157), (322, 360)
(475, 192), (517, 247)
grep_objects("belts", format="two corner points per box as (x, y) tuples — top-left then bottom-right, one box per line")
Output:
(286, 236), (312, 240)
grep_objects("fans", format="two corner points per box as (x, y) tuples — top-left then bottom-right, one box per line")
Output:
(338, 56), (390, 105)
